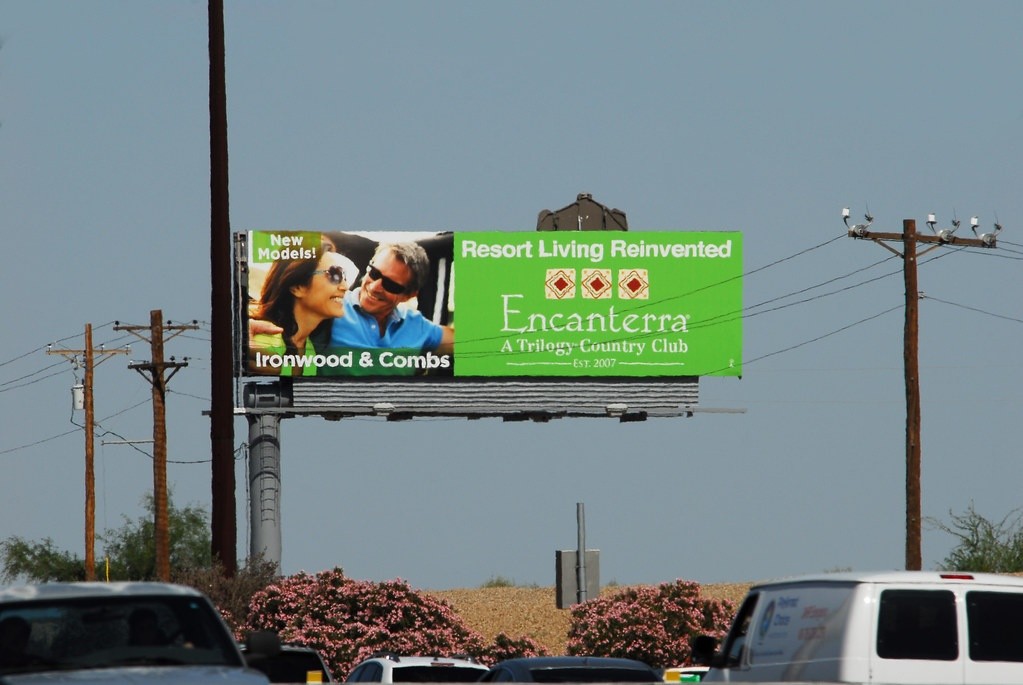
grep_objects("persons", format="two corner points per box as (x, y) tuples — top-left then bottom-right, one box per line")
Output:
(248, 233), (455, 376)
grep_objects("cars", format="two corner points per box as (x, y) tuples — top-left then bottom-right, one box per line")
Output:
(0, 579), (272, 685)
(476, 657), (665, 685)
(239, 643), (335, 685)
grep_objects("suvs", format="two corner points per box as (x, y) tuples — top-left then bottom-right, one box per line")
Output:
(344, 651), (491, 685)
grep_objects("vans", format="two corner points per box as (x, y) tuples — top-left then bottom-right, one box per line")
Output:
(690, 569), (1023, 685)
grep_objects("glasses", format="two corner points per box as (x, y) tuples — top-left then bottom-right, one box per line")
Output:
(369, 264), (413, 294)
(312, 266), (347, 285)
